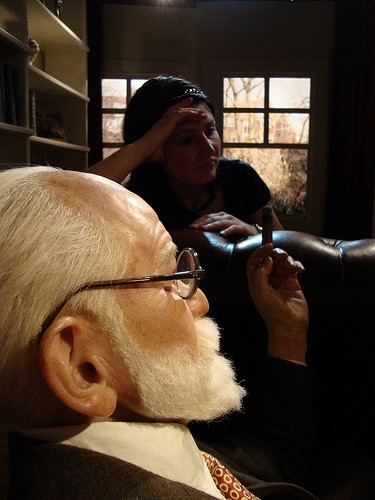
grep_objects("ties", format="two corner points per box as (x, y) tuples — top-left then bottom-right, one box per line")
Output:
(200, 451), (261, 500)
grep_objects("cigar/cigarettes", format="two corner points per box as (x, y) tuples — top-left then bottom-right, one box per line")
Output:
(261, 206), (273, 283)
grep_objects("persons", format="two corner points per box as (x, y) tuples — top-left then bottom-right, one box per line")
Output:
(0, 166), (318, 500)
(84, 76), (283, 236)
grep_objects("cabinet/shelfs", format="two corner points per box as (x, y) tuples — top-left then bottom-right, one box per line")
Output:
(0, 0), (89, 172)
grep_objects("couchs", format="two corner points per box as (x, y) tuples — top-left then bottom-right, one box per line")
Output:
(169, 229), (375, 496)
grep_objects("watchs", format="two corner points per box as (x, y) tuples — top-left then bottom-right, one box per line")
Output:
(252, 223), (263, 233)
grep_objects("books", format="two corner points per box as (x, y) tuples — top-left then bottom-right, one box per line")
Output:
(0, 62), (18, 121)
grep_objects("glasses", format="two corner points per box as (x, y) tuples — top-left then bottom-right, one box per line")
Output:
(34, 247), (206, 346)
